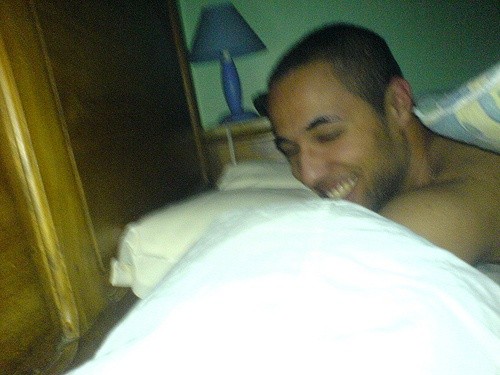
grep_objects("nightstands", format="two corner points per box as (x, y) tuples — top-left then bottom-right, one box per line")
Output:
(204, 116), (287, 174)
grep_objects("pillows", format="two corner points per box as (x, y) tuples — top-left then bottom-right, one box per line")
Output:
(69, 157), (500, 372)
(412, 61), (500, 156)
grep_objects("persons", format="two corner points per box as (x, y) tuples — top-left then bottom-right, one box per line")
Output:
(266, 20), (500, 271)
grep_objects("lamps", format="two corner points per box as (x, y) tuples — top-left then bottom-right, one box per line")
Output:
(189, 3), (268, 126)
